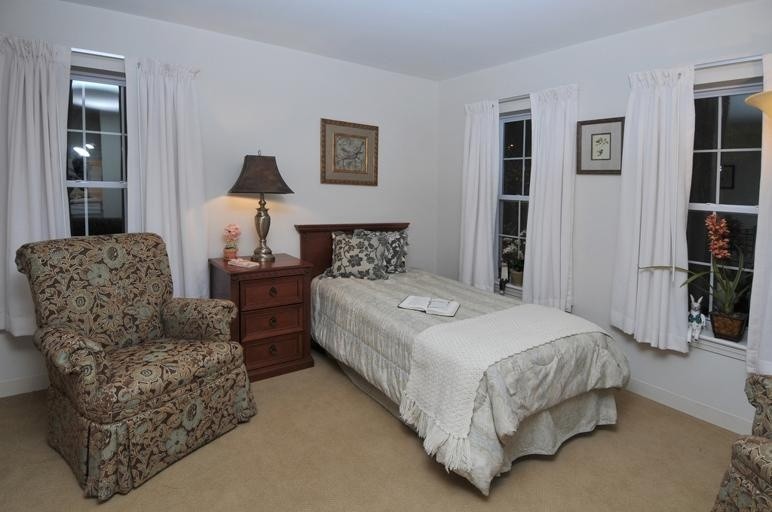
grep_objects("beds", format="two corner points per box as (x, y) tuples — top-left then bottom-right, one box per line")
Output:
(296, 222), (631, 500)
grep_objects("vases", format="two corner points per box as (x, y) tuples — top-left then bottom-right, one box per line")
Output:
(710, 311), (747, 342)
(224, 248), (238, 260)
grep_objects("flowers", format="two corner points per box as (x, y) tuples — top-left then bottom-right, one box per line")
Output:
(221, 225), (243, 259)
(638, 213), (751, 314)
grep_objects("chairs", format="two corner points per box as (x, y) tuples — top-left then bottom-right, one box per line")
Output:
(16, 232), (257, 505)
(708, 372), (771, 512)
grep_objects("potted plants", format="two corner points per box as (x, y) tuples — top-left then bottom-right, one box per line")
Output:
(501, 230), (526, 287)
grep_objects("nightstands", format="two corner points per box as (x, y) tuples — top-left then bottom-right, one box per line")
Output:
(207, 253), (314, 383)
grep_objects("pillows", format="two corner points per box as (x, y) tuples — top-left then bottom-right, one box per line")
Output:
(354, 229), (408, 272)
(329, 230), (389, 280)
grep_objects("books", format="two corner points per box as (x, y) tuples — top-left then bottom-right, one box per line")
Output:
(228, 258), (260, 268)
(397, 295), (461, 317)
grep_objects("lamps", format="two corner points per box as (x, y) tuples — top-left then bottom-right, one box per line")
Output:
(230, 154), (293, 262)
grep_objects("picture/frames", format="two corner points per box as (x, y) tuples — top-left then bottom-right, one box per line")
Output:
(572, 117), (630, 175)
(319, 117), (382, 186)
